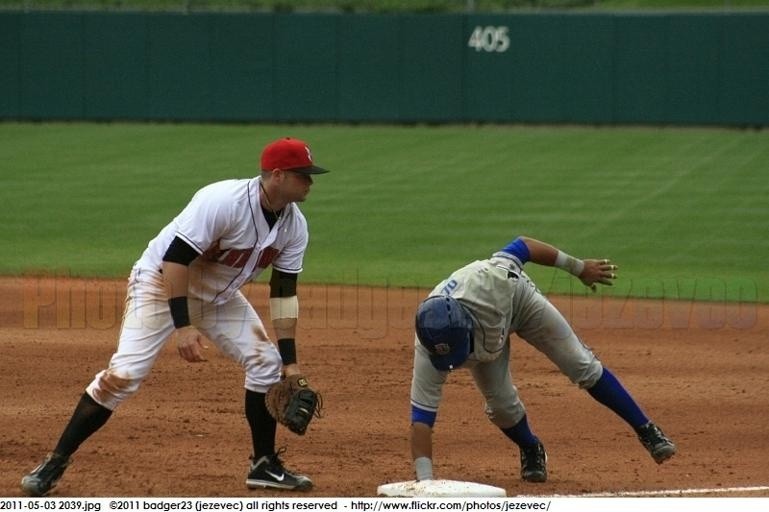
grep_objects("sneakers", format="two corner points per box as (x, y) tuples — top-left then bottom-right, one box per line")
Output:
(638, 421), (676, 464)
(520, 435), (547, 481)
(245, 455), (313, 491)
(21, 452), (69, 496)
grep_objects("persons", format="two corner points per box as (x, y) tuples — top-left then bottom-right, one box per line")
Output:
(17, 136), (331, 496)
(409, 235), (678, 482)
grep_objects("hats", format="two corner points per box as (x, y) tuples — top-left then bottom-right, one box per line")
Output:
(260, 137), (330, 174)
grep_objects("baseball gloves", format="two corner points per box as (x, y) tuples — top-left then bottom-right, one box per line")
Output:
(265, 373), (319, 435)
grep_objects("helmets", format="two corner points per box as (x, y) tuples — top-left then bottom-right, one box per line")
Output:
(415, 295), (473, 372)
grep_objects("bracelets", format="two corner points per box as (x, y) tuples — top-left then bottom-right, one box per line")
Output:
(554, 252), (587, 278)
(414, 457), (435, 482)
(276, 338), (298, 365)
(167, 295), (192, 329)
